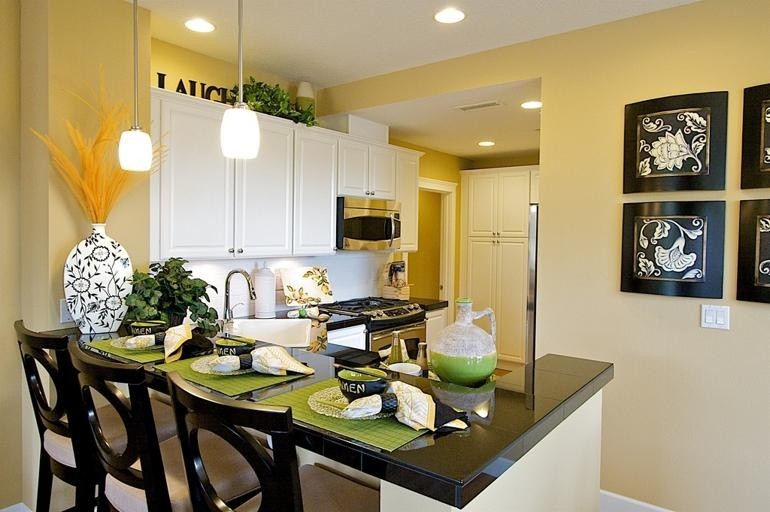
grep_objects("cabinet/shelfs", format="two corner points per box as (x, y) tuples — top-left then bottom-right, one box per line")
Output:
(293, 115), (426, 259)
(458, 239), (531, 373)
(457, 166), (532, 240)
(151, 86), (294, 262)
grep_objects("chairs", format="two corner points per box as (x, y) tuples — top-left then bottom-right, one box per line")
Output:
(67, 333), (300, 511)
(13, 318), (184, 512)
(166, 370), (381, 512)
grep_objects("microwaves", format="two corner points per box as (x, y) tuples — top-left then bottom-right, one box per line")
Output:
(337, 195), (402, 251)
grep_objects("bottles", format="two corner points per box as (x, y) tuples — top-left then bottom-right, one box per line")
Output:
(392, 260), (407, 287)
(416, 342), (428, 379)
(386, 330), (402, 363)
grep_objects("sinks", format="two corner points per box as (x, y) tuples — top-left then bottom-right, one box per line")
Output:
(220, 318), (312, 348)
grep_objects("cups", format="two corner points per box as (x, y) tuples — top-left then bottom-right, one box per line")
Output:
(387, 363), (422, 377)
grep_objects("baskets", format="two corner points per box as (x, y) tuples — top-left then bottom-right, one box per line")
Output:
(382, 262), (411, 300)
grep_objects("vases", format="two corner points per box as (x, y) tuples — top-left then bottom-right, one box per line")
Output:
(63, 222), (135, 334)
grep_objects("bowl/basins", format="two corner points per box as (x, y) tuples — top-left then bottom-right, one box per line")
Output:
(337, 366), (386, 402)
(130, 320), (166, 335)
(215, 338), (255, 357)
(383, 284), (410, 301)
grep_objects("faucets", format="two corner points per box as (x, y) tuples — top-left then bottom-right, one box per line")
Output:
(223, 268), (256, 320)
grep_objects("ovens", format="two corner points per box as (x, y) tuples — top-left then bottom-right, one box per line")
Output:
(369, 325), (427, 360)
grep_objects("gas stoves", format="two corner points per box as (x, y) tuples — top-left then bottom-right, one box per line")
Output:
(319, 296), (426, 332)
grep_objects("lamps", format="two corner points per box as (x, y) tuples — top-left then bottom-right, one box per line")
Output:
(219, 2), (261, 161)
(116, 0), (154, 172)
